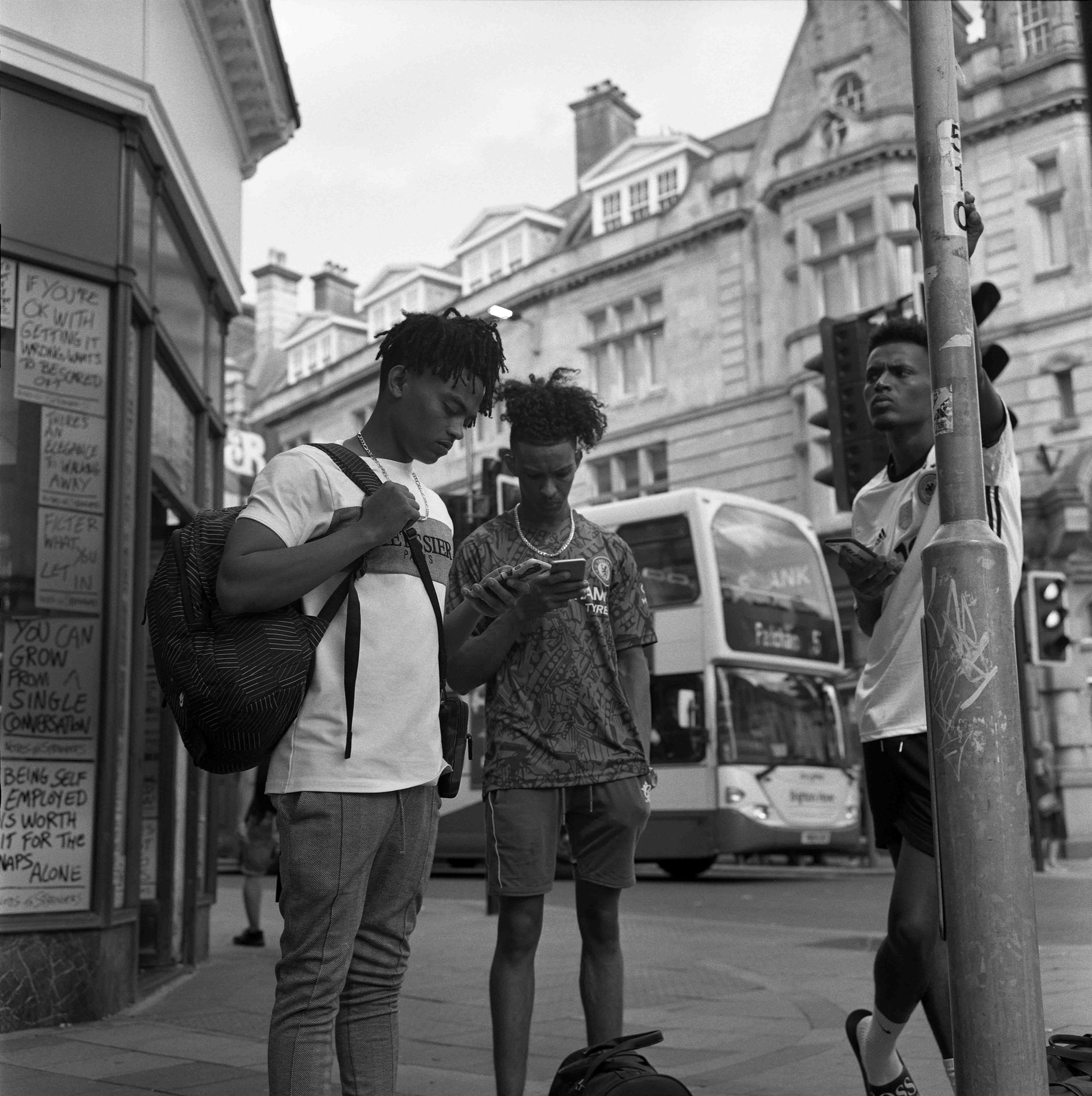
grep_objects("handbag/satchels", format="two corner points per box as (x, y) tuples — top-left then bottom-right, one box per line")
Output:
(1046, 1034), (1092, 1096)
(548, 1029), (693, 1096)
(1038, 792), (1061, 819)
(436, 697), (474, 799)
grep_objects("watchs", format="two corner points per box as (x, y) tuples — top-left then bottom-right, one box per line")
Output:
(648, 766), (658, 789)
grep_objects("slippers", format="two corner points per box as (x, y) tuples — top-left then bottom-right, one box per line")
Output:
(846, 1009), (920, 1096)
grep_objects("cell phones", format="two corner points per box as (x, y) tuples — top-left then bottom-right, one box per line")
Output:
(824, 537), (898, 576)
(550, 558), (586, 583)
(470, 558), (552, 598)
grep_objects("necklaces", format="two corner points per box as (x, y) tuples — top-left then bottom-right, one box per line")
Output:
(357, 431), (430, 523)
(511, 501), (576, 558)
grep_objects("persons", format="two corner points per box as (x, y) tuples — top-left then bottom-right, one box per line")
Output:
(1033, 738), (1069, 871)
(444, 363), (660, 1095)
(836, 181), (1024, 1096)
(213, 300), (507, 1096)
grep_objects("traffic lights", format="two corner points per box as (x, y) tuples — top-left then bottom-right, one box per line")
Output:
(1023, 572), (1073, 665)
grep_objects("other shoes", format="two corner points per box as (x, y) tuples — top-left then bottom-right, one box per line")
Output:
(234, 929), (264, 946)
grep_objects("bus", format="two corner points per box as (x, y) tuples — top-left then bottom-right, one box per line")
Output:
(430, 487), (865, 881)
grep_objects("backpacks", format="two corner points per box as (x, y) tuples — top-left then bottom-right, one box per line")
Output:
(141, 443), (385, 776)
